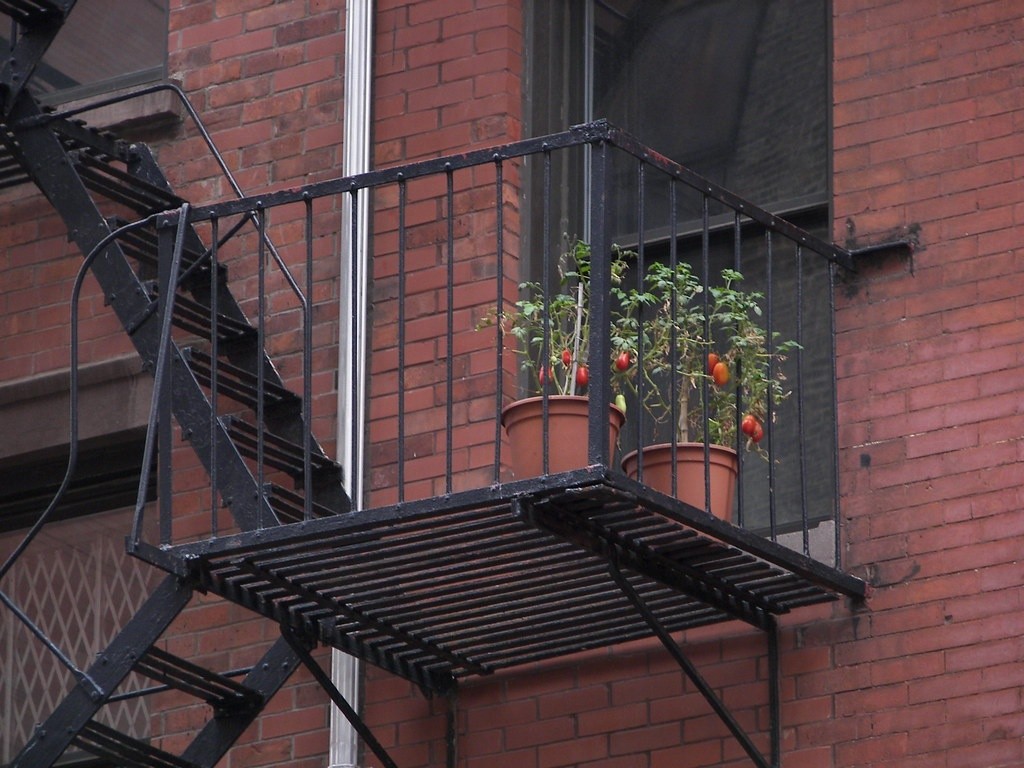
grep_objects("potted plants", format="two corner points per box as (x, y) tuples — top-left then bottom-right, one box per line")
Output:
(472, 235), (717, 481)
(619, 263), (804, 548)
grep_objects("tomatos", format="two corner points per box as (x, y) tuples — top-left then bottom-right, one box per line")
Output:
(708, 352), (729, 387)
(742, 414), (763, 442)
(539, 348), (630, 415)
